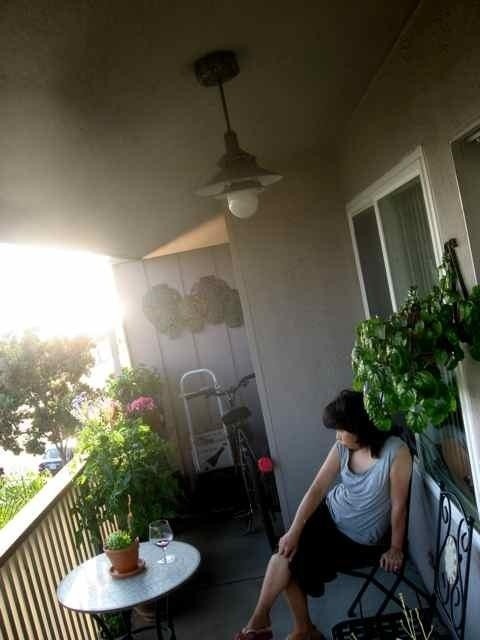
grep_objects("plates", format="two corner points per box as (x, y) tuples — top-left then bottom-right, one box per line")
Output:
(110, 558), (145, 578)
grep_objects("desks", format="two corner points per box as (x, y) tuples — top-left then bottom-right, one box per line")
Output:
(54, 539), (203, 639)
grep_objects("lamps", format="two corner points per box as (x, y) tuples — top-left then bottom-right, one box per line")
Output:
(188, 53), (284, 223)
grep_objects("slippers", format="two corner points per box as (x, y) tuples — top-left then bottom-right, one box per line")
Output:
(235, 627), (273, 640)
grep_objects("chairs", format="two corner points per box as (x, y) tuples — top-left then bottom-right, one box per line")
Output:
(330, 425), (474, 639)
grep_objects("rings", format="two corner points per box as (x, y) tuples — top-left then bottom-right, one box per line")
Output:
(393, 566), (398, 568)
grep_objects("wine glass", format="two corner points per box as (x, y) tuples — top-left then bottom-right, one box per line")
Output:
(149, 519), (177, 565)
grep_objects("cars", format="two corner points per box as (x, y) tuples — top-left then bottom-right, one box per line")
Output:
(38, 444), (74, 477)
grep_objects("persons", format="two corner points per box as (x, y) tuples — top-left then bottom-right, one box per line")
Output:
(233, 389), (413, 640)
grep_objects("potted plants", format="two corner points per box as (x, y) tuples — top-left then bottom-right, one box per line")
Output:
(102, 529), (146, 578)
(342, 241), (479, 434)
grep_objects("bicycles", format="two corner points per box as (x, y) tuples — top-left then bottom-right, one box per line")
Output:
(184, 372), (281, 549)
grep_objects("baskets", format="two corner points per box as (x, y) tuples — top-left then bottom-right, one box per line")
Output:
(328, 606), (456, 640)
